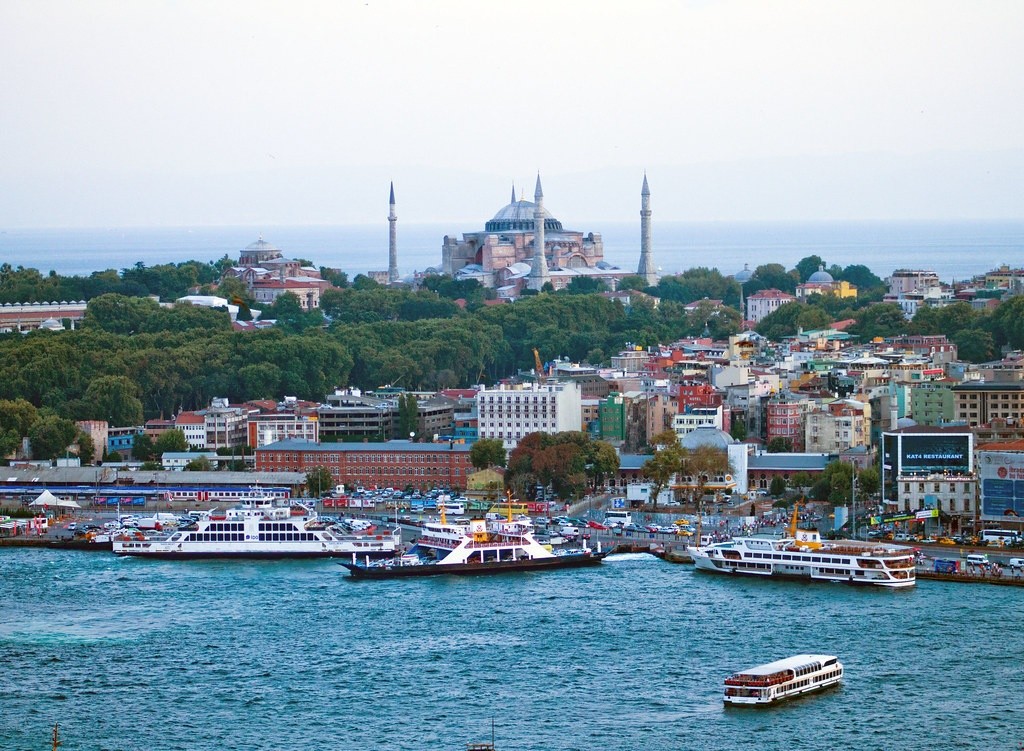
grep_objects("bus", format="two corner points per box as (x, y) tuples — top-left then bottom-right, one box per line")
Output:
(979, 529), (1024, 545)
(436, 503), (465, 516)
(603, 511), (631, 523)
(486, 503), (529, 516)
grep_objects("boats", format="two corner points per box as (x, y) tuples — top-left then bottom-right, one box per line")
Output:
(687, 529), (916, 588)
(649, 540), (694, 563)
(112, 481), (401, 561)
(722, 654), (843, 708)
(336, 489), (614, 578)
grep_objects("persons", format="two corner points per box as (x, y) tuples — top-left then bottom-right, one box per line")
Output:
(970, 560), (1002, 580)
(717, 510), (816, 543)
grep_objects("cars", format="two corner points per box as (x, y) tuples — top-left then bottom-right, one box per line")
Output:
(67, 511), (207, 544)
(613, 520), (694, 537)
(893, 533), (979, 545)
(534, 525), (590, 544)
(331, 485), (491, 514)
(536, 516), (607, 531)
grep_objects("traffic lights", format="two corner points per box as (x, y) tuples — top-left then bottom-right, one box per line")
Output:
(399, 508), (405, 513)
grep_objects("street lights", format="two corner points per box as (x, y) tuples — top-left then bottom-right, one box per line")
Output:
(155, 461), (161, 522)
(848, 455), (858, 540)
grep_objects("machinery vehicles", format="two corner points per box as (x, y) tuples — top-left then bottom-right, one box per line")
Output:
(787, 495), (807, 537)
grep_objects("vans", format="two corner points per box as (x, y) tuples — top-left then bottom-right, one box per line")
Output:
(967, 555), (990, 566)
(1011, 558), (1024, 569)
